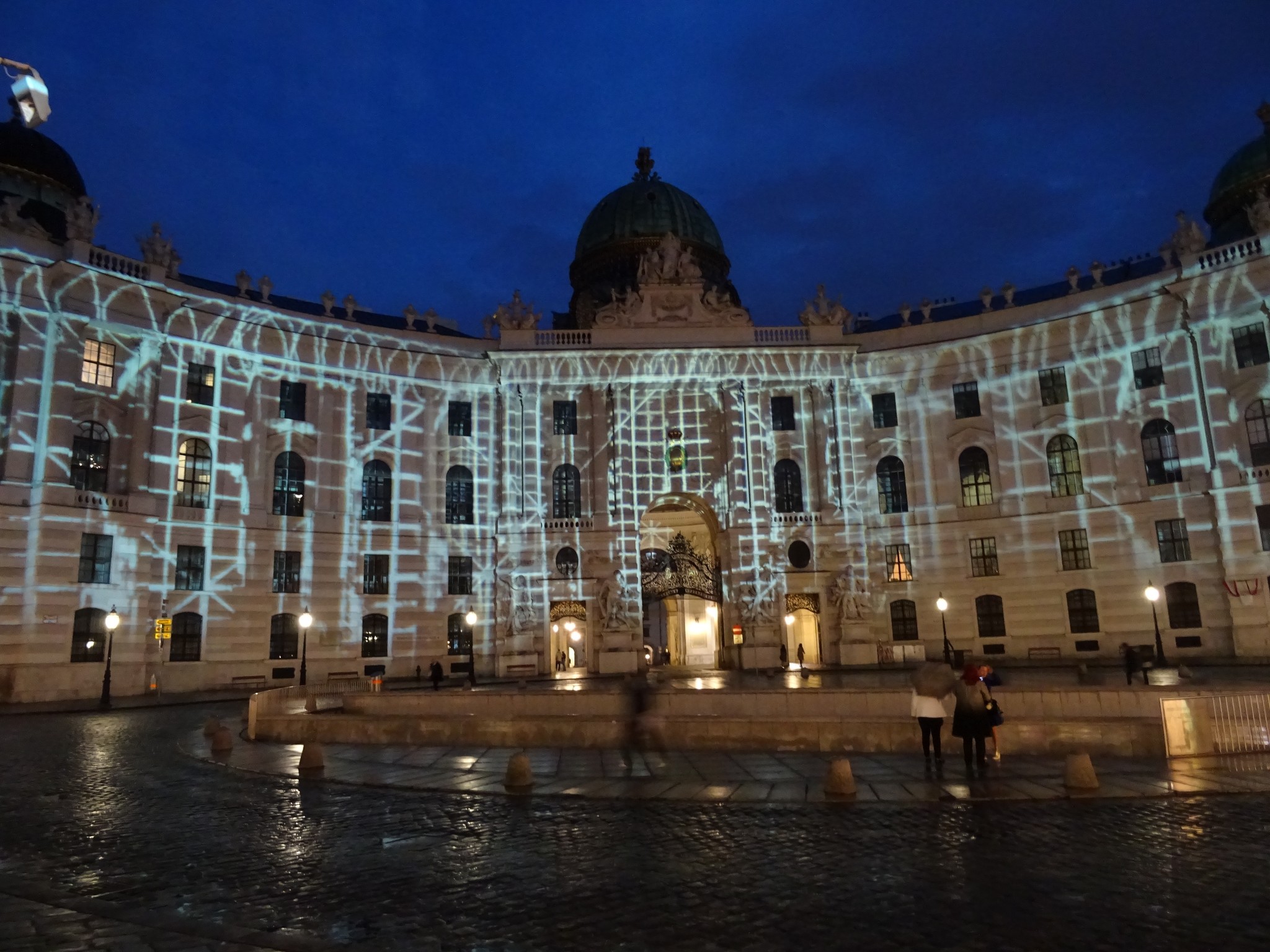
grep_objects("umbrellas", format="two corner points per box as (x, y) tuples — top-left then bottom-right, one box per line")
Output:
(909, 661), (958, 700)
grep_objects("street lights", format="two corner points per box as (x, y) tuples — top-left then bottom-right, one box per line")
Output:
(300, 606), (313, 686)
(936, 592), (950, 662)
(465, 605), (478, 684)
(1145, 579), (1170, 667)
(97, 604), (121, 710)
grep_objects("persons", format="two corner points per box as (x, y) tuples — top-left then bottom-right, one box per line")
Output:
(739, 569), (778, 625)
(556, 648), (562, 671)
(613, 231), (725, 311)
(951, 664), (992, 773)
(492, 293), (542, 330)
(1120, 643), (1143, 685)
(833, 564), (867, 621)
(911, 688), (948, 764)
(656, 647), (664, 665)
(801, 287), (849, 326)
(429, 658), (443, 691)
(976, 666), (1003, 762)
(497, 575), (530, 635)
(599, 570), (633, 630)
(664, 649), (671, 665)
(779, 644), (787, 668)
(797, 644), (805, 668)
(561, 651), (566, 671)
